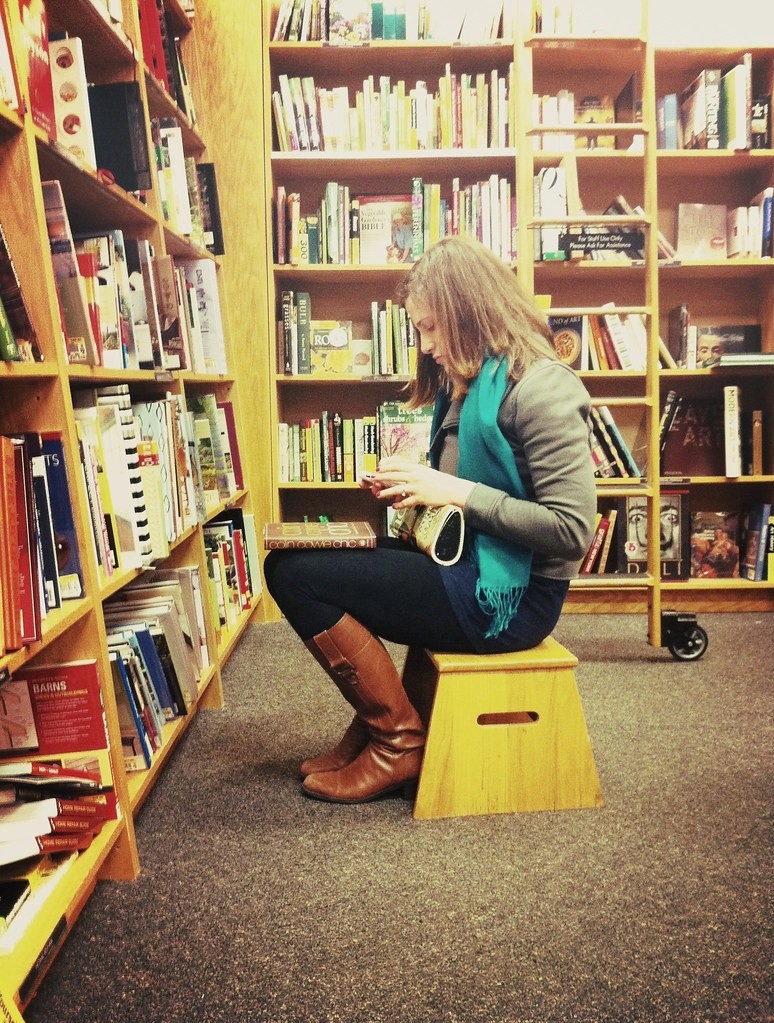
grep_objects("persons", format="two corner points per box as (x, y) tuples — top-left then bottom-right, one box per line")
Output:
(267, 2), (772, 579)
(264, 230), (596, 802)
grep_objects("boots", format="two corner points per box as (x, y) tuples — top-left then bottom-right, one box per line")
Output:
(300, 715), (372, 778)
(302, 612), (427, 803)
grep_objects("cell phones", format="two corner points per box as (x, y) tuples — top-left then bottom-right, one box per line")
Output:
(359, 471), (406, 497)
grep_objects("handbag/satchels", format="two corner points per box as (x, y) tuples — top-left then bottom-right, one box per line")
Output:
(390, 504), (465, 566)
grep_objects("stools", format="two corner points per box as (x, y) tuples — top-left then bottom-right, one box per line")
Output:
(402, 633), (608, 821)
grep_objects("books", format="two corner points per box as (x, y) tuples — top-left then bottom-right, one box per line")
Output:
(0, 0), (264, 949)
(264, 519), (376, 549)
(390, 505), (465, 566)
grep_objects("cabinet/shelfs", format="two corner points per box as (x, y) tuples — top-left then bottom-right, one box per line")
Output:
(0, 0), (273, 1023)
(262, 0), (774, 625)
